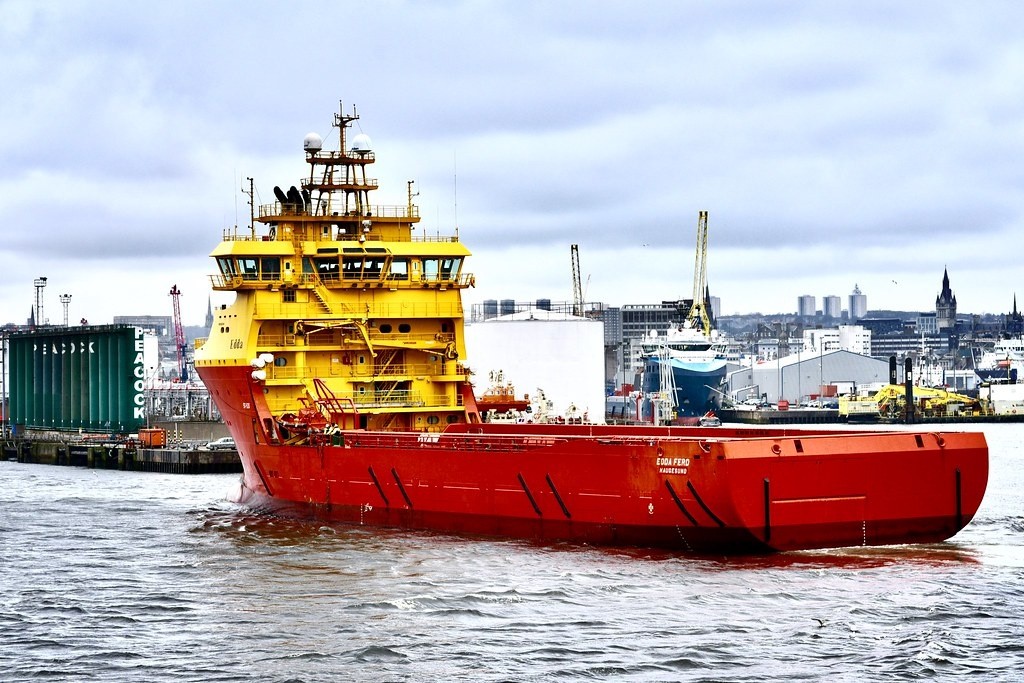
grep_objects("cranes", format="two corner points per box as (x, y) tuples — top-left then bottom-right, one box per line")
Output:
(170, 283), (189, 387)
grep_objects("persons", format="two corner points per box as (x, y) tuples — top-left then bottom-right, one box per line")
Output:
(324, 424), (341, 445)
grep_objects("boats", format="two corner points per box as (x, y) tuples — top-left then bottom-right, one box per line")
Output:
(635, 328), (729, 422)
(187, 97), (996, 556)
